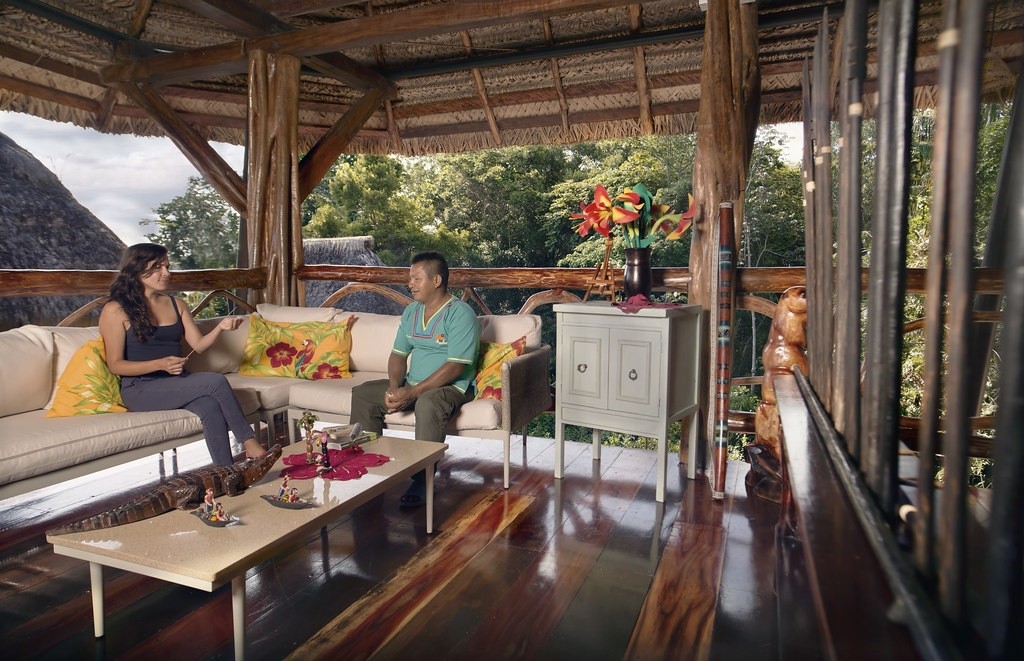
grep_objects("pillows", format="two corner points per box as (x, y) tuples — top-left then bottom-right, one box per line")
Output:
(43, 333), (131, 419)
(468, 336), (529, 405)
(237, 310), (358, 381)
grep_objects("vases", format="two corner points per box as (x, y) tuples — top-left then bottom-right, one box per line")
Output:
(624, 247), (653, 301)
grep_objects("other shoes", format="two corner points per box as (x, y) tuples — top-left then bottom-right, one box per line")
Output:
(399, 474), (436, 506)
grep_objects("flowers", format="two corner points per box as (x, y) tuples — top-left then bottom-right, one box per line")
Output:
(571, 172), (699, 250)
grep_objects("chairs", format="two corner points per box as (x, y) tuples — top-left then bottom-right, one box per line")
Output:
(181, 301), (344, 454)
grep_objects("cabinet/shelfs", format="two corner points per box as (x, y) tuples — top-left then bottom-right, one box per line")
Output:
(554, 299), (704, 499)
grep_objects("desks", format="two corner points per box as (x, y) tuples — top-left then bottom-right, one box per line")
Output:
(45, 432), (449, 661)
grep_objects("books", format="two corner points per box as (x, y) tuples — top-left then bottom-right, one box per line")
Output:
(317, 424), (377, 450)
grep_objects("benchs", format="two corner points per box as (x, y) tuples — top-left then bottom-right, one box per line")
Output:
(287, 311), (553, 491)
(0, 324), (262, 503)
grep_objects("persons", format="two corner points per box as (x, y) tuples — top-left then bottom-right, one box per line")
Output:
(204, 488), (228, 521)
(98, 243), (267, 465)
(277, 475), (299, 503)
(350, 252), (480, 504)
